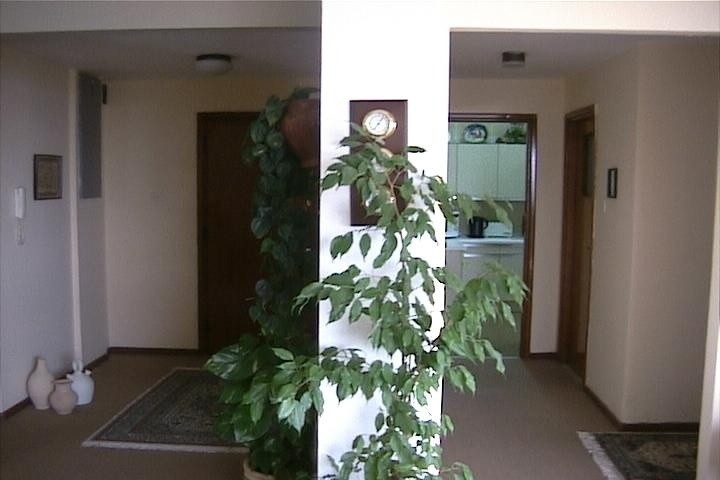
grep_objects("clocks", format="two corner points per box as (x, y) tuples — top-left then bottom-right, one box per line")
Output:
(362, 109), (398, 139)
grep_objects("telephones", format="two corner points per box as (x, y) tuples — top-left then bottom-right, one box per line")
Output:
(14, 187), (27, 219)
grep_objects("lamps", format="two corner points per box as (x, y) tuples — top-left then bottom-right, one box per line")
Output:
(196, 53), (232, 75)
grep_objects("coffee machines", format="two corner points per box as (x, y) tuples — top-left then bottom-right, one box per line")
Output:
(446, 212), (460, 237)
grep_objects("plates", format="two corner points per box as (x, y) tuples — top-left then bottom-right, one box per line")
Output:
(463, 124), (487, 143)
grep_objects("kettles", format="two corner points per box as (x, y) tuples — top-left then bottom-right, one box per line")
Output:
(469, 216), (489, 236)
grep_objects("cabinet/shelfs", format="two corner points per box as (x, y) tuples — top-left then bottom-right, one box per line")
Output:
(446, 238), (525, 306)
(448, 143), (526, 203)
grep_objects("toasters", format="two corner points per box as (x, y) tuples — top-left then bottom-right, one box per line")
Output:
(482, 220), (514, 238)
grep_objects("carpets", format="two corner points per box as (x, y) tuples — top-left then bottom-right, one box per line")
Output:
(81, 367), (248, 454)
(576, 430), (697, 480)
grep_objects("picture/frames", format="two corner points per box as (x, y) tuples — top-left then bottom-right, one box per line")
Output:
(33, 154), (63, 200)
(608, 168), (617, 198)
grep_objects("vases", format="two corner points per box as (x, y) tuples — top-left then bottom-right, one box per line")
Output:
(27, 358), (95, 416)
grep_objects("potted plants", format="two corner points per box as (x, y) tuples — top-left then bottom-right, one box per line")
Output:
(198, 336), (317, 479)
(237, 85), (319, 347)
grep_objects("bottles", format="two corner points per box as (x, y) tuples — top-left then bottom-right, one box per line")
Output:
(27, 356), (56, 412)
(47, 380), (79, 417)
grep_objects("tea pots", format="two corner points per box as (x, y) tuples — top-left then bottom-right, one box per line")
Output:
(66, 358), (95, 407)
